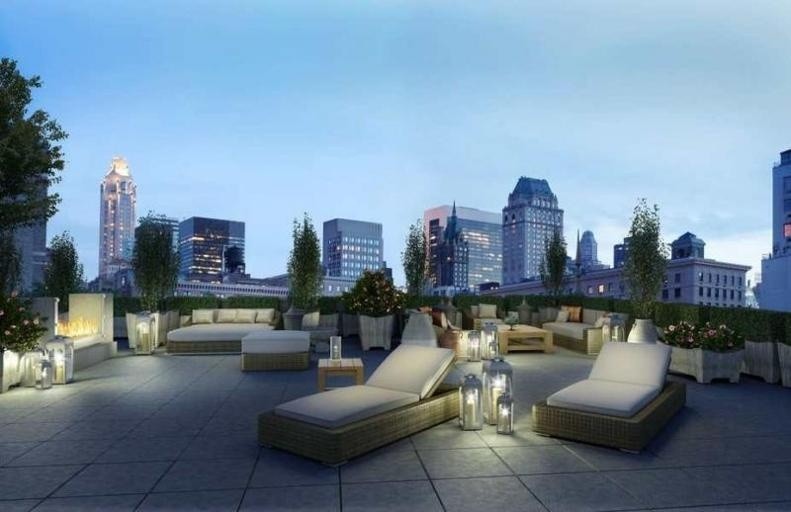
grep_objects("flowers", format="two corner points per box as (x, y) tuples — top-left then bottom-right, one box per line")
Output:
(661, 322), (746, 350)
(341, 269), (409, 316)
(0, 289), (51, 354)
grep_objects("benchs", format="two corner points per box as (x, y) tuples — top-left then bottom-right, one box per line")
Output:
(241, 329), (310, 371)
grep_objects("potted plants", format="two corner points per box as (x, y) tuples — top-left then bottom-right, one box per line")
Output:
(125, 209), (180, 349)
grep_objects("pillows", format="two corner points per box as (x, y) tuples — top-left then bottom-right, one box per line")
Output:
(192, 307), (276, 324)
(555, 305), (582, 322)
(471, 303), (498, 319)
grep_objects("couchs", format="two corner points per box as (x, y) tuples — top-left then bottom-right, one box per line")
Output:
(543, 309), (628, 352)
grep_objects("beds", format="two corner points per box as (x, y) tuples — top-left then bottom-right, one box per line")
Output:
(168, 303), (280, 356)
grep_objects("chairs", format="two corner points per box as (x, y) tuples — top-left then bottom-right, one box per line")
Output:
(464, 309), (501, 329)
